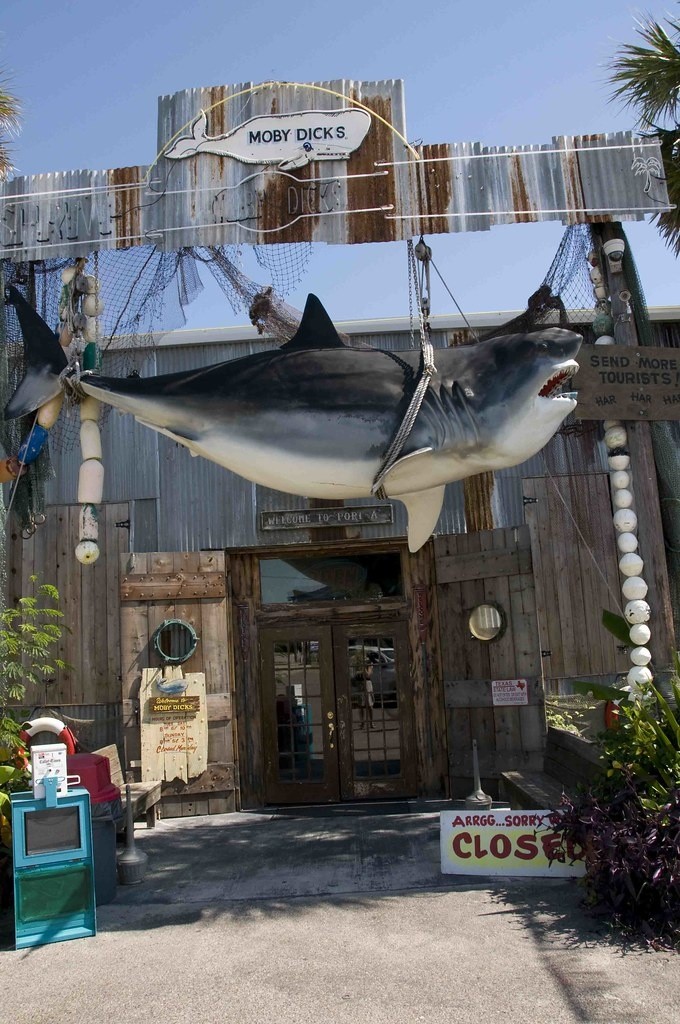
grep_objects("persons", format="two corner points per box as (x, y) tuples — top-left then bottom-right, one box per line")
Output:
(354, 657), (376, 729)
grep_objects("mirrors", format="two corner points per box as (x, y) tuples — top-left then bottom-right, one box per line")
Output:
(468, 600), (508, 643)
(152, 618), (200, 665)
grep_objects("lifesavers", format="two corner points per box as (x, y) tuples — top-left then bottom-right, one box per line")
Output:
(15, 717), (76, 775)
(605, 685), (653, 731)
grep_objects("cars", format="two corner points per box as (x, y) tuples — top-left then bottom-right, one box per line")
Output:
(347, 644), (397, 709)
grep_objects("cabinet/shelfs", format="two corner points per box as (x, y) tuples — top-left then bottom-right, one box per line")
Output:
(9, 786), (98, 950)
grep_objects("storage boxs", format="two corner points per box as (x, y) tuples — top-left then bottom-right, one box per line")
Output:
(31, 741), (68, 798)
(68, 752), (111, 793)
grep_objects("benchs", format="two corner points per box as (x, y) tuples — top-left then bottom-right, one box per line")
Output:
(91, 743), (162, 834)
(498, 726), (614, 813)
